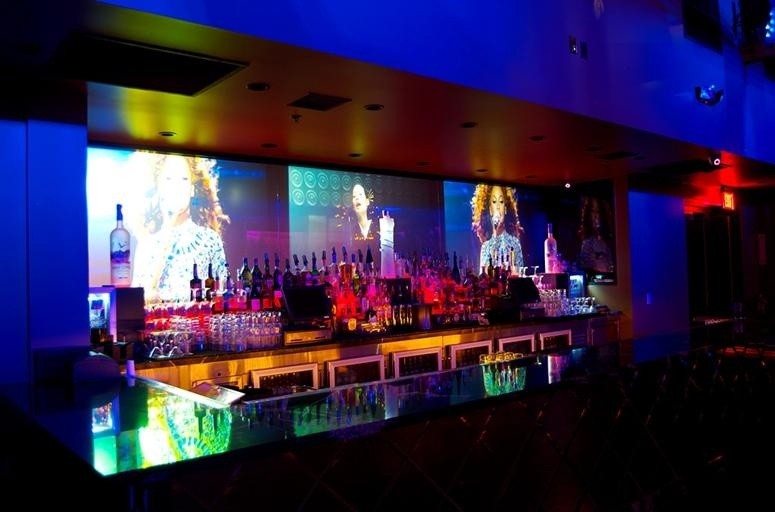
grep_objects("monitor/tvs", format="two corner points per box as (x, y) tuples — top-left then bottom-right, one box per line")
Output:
(507, 277), (542, 305)
(280, 285), (332, 323)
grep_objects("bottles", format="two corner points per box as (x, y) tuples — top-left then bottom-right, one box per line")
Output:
(114, 428), (140, 473)
(109, 204), (134, 288)
(544, 223), (561, 272)
(89, 297), (107, 328)
(193, 349), (542, 441)
(546, 352), (565, 385)
(145, 245), (517, 314)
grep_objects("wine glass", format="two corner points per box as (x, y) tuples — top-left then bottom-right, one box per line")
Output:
(362, 298), (490, 337)
(145, 312), (285, 363)
(517, 265), (605, 316)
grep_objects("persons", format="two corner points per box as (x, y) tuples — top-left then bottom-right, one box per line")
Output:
(579, 192), (613, 274)
(343, 180), (397, 250)
(120, 152), (231, 300)
(471, 183), (525, 274)
